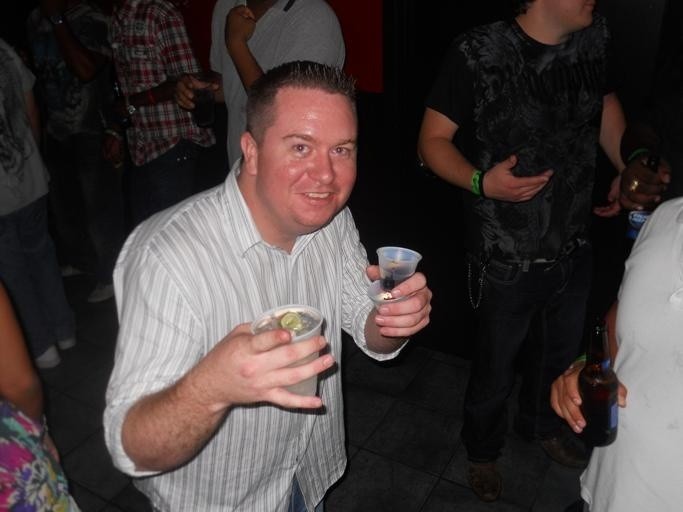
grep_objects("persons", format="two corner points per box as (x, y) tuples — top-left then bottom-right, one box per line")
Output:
(0, 0), (382, 368)
(0, 281), (82, 512)
(417, 0), (683, 512)
(103, 61), (432, 511)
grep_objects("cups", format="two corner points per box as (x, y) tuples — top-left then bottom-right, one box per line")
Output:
(190, 71), (216, 129)
(251, 304), (323, 395)
(369, 244), (421, 312)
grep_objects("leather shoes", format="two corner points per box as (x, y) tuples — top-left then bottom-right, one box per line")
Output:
(541, 436), (590, 468)
(465, 465), (502, 500)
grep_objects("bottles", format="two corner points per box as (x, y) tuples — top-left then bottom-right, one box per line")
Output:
(627, 153), (661, 248)
(111, 82), (130, 127)
(577, 320), (619, 448)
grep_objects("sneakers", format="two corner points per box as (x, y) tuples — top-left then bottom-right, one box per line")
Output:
(60, 265), (83, 276)
(34, 337), (75, 369)
(87, 281), (115, 303)
